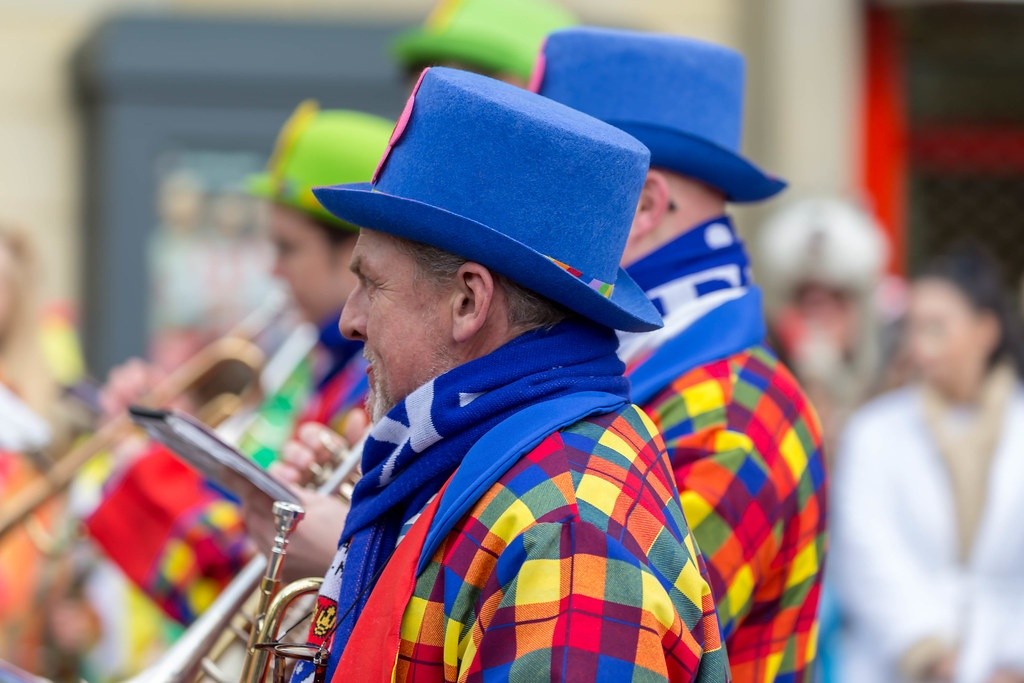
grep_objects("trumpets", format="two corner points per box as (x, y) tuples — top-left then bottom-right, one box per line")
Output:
(238, 501), (324, 683)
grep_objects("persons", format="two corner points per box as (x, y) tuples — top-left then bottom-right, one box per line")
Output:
(239, 25), (830, 682)
(100, 100), (390, 683)
(297, 67), (735, 683)
(0, 192), (1024, 683)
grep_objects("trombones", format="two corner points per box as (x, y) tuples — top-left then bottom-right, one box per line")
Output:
(124, 422), (370, 682)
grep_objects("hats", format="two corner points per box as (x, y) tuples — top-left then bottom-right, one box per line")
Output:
(766, 198), (892, 298)
(532, 27), (786, 205)
(391, 0), (578, 87)
(312, 68), (664, 333)
(240, 98), (396, 231)
